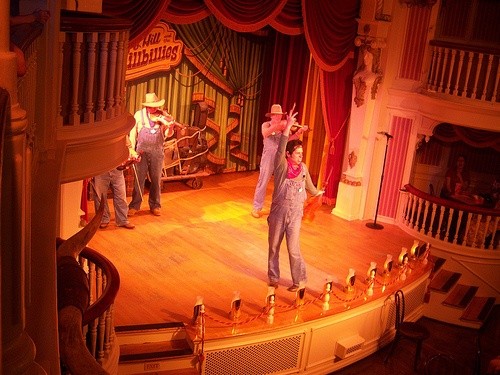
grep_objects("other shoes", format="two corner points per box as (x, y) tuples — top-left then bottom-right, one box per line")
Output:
(101, 223), (108, 228)
(128, 209), (137, 215)
(118, 223), (135, 228)
(288, 285), (299, 291)
(270, 283), (278, 289)
(252, 209), (260, 218)
(150, 208), (161, 216)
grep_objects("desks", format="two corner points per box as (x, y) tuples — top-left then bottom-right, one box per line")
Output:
(447, 192), (487, 211)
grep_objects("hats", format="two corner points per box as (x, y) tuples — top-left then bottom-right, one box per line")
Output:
(142, 93), (165, 107)
(265, 105), (287, 117)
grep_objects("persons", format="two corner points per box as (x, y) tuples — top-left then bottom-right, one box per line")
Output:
(252, 104), (308, 218)
(130, 93), (174, 216)
(267, 108), (326, 291)
(361, 45), (380, 72)
(439, 154), (470, 198)
(95, 134), (141, 229)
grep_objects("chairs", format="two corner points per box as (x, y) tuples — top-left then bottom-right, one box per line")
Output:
(429, 183), (435, 197)
(384, 290), (430, 372)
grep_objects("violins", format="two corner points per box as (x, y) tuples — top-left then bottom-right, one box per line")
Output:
(301, 180), (329, 223)
(117, 150), (141, 171)
(151, 109), (186, 131)
(274, 120), (311, 132)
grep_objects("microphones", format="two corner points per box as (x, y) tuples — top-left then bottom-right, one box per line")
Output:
(383, 132), (393, 138)
